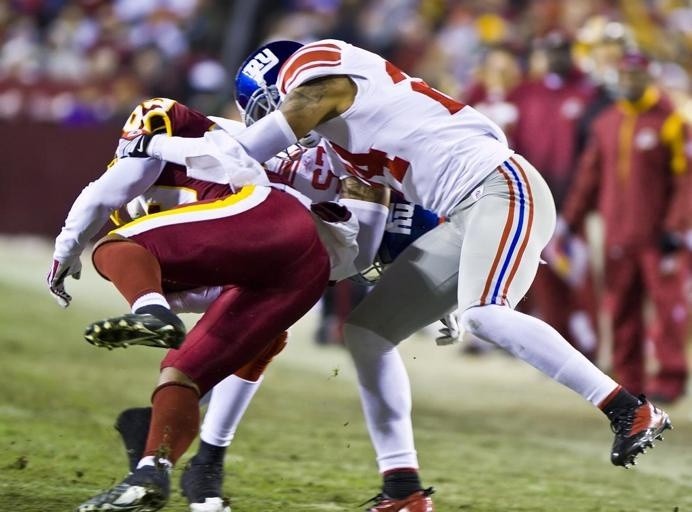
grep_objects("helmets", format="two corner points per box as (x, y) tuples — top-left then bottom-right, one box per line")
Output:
(232, 40), (318, 145)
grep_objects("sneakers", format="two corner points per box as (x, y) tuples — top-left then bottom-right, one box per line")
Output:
(179, 456), (234, 512)
(76, 464), (171, 511)
(609, 391), (680, 468)
(369, 482), (435, 512)
(83, 303), (186, 350)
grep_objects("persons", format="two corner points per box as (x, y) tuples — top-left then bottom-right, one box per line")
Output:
(316, 1), (691, 404)
(113, 141), (391, 470)
(46, 97), (329, 511)
(207, 39), (677, 512)
(0, 0), (409, 126)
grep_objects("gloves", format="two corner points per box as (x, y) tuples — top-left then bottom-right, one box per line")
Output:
(118, 136), (155, 161)
(435, 309), (463, 346)
(45, 257), (82, 309)
